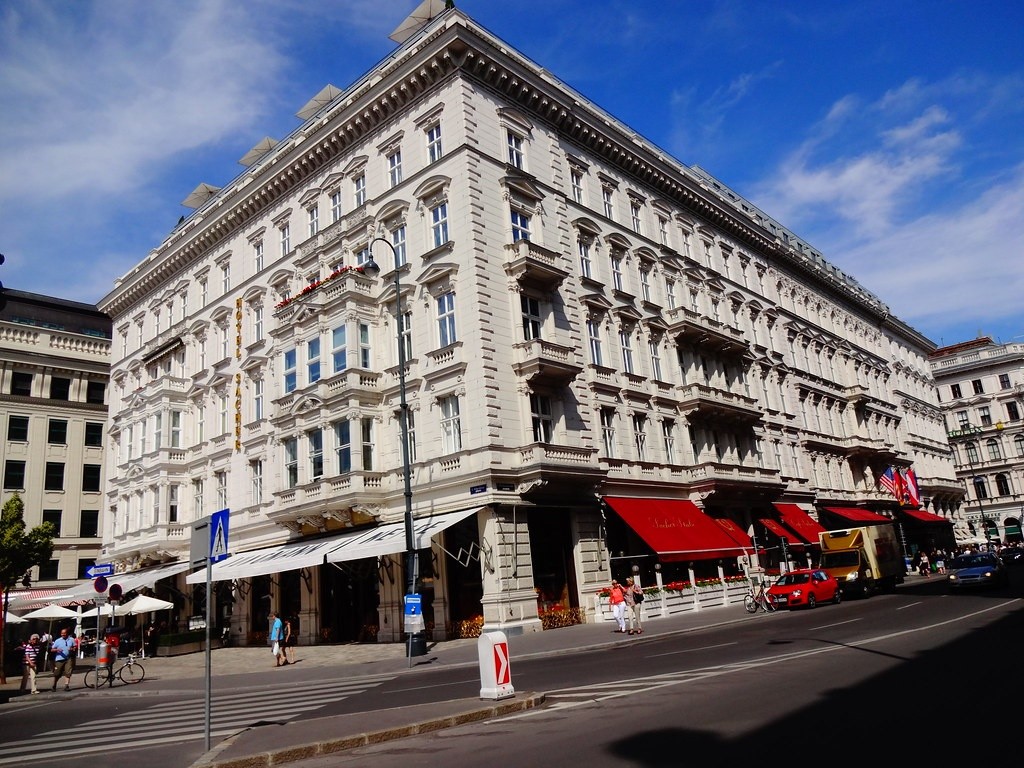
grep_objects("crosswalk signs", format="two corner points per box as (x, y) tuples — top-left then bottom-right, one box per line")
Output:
(207, 507), (232, 565)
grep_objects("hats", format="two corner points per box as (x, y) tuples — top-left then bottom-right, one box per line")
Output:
(270, 612), (278, 617)
(626, 577), (634, 582)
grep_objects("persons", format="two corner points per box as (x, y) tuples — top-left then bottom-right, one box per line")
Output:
(159, 619), (169, 634)
(139, 621), (158, 657)
(270, 612), (296, 667)
(947, 551), (952, 571)
(42, 626), (76, 692)
(609, 577), (643, 634)
(912, 547), (947, 578)
(74, 633), (96, 658)
(14, 633), (41, 694)
(988, 540), (1024, 556)
(950, 544), (985, 560)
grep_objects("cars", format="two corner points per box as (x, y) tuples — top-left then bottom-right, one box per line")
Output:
(943, 546), (1024, 592)
(764, 567), (842, 610)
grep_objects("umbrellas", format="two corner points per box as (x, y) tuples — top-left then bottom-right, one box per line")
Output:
(1, 610), (29, 624)
(82, 594), (173, 659)
(20, 603), (82, 673)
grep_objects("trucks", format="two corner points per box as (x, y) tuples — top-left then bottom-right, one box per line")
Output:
(817, 523), (907, 596)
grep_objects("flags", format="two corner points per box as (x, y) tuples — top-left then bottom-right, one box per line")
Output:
(880, 468), (920, 506)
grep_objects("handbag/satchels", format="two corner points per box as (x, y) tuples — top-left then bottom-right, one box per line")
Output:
(272, 640), (280, 657)
(633, 584), (644, 604)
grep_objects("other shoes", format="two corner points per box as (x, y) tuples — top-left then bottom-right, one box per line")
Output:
(51, 687), (56, 692)
(31, 691), (40, 694)
(629, 629), (634, 634)
(638, 629), (640, 633)
(64, 686), (72, 691)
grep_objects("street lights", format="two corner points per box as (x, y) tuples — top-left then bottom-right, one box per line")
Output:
(364, 236), (431, 656)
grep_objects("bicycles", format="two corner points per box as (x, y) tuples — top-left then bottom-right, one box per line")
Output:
(84, 651), (146, 689)
(742, 580), (780, 615)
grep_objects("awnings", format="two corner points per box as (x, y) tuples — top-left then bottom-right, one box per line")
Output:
(956, 536), (996, 544)
(602, 497), (767, 562)
(186, 504), (488, 585)
(755, 503), (830, 552)
(2, 561), (190, 611)
(824, 507), (894, 525)
(902, 511), (948, 527)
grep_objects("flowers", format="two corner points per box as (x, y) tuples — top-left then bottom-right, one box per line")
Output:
(596, 575), (749, 601)
(767, 569), (780, 577)
